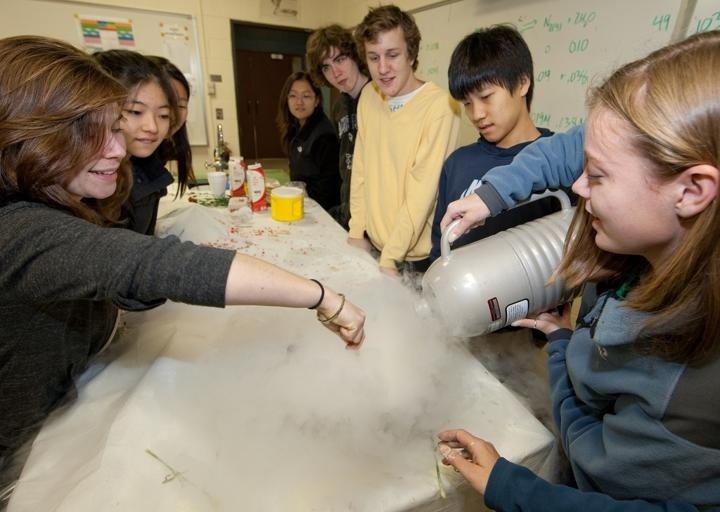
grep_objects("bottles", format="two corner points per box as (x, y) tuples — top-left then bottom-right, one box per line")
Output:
(228, 156), (246, 197)
(246, 164), (266, 212)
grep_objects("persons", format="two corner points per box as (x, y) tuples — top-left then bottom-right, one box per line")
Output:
(143, 53), (197, 235)
(304, 24), (372, 242)
(277, 70), (341, 222)
(0, 36), (363, 511)
(87, 48), (174, 235)
(425, 25), (587, 483)
(436, 428), (698, 512)
(346, 6), (462, 291)
(440, 29), (719, 512)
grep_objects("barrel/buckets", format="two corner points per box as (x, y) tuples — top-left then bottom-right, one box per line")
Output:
(409, 190), (587, 340)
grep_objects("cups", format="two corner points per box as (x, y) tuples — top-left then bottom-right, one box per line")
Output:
(208, 172), (227, 199)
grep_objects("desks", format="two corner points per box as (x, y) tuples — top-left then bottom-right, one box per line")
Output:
(6, 198), (557, 512)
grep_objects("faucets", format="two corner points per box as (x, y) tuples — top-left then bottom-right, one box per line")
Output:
(216, 123), (223, 161)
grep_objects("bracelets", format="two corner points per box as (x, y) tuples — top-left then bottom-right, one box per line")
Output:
(308, 278), (324, 310)
(317, 291), (345, 322)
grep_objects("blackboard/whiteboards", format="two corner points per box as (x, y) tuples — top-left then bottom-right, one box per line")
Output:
(0, 0), (210, 148)
(346, 0), (720, 154)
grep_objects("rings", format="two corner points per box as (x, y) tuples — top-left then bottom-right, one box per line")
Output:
(533, 319), (537, 329)
